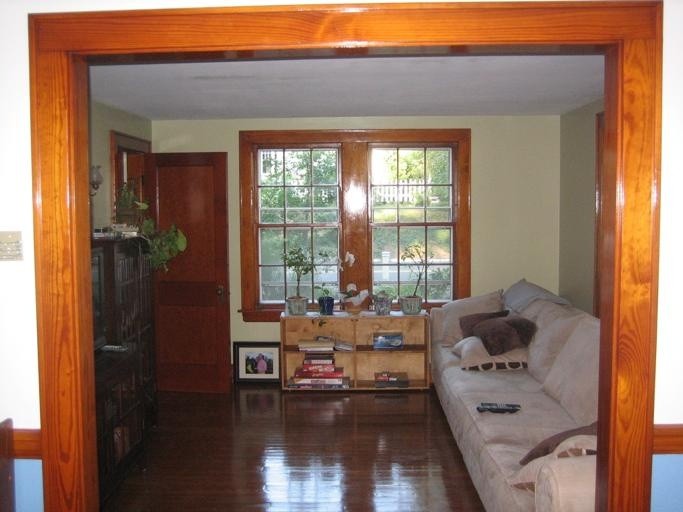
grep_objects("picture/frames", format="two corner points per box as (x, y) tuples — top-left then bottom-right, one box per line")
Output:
(232, 341), (281, 386)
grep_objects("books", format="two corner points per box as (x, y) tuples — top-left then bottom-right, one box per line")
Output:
(289, 336), (353, 388)
(375, 372), (409, 388)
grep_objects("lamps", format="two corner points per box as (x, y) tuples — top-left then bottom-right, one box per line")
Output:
(88, 164), (104, 209)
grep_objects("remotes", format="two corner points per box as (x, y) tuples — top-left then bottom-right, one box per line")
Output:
(481, 403), (520, 407)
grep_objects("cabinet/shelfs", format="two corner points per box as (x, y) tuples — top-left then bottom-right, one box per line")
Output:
(278, 309), (431, 393)
(89, 230), (161, 511)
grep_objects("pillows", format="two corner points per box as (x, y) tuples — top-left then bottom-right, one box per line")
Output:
(518, 420), (597, 466)
(507, 433), (597, 497)
(438, 281), (537, 372)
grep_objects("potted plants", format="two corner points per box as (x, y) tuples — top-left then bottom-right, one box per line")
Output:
(110, 174), (187, 277)
(312, 282), (335, 316)
(278, 246), (320, 316)
(398, 240), (436, 315)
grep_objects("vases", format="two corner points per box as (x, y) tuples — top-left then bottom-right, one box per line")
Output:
(374, 300), (391, 317)
(343, 302), (362, 315)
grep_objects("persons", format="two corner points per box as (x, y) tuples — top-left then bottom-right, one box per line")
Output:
(257, 353), (267, 374)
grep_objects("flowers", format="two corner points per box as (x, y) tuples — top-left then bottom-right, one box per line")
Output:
(369, 285), (398, 305)
(335, 251), (369, 306)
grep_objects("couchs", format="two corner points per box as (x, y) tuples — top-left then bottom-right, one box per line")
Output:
(428, 276), (600, 512)
